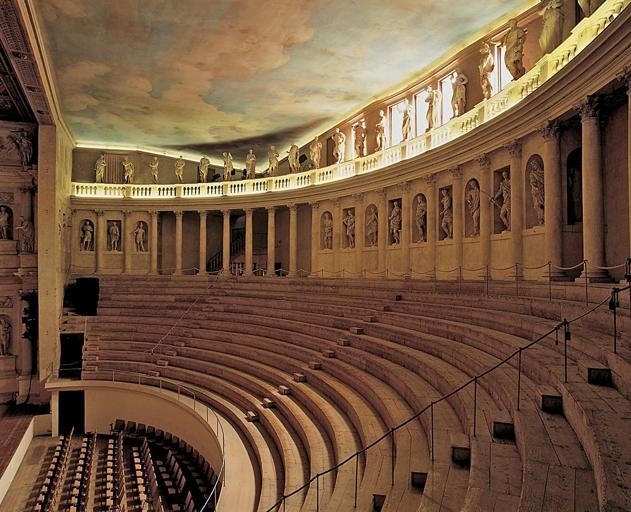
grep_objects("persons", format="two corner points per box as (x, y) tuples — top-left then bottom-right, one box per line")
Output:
(287, 144), (300, 173)
(268, 146), (279, 177)
(0, 206), (9, 239)
(198, 154), (209, 183)
(451, 20), (527, 119)
(309, 136), (322, 170)
(222, 152), (233, 181)
(389, 189), (453, 245)
(121, 156), (134, 183)
(401, 86), (439, 142)
(537, 2), (566, 52)
(83, 220), (146, 252)
(353, 109), (386, 158)
(465, 159), (544, 237)
(323, 205), (379, 249)
(246, 149), (256, 179)
(331, 128), (346, 163)
(149, 156), (159, 184)
(0, 318), (11, 356)
(94, 154), (107, 183)
(175, 156), (186, 184)
(5, 132), (33, 166)
(15, 216), (34, 253)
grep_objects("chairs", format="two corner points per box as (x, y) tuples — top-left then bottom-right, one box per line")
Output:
(31, 419), (222, 512)
